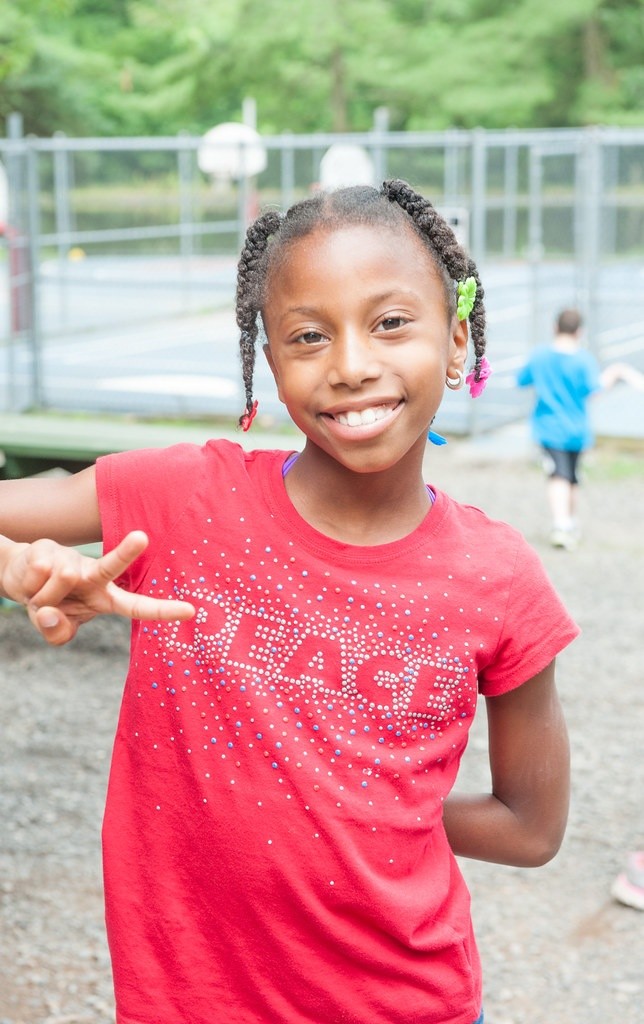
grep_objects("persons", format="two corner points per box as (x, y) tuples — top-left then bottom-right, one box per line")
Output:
(1, 178), (576, 1023)
(519, 307), (623, 550)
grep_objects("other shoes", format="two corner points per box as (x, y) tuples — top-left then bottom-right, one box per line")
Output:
(549, 534), (567, 548)
